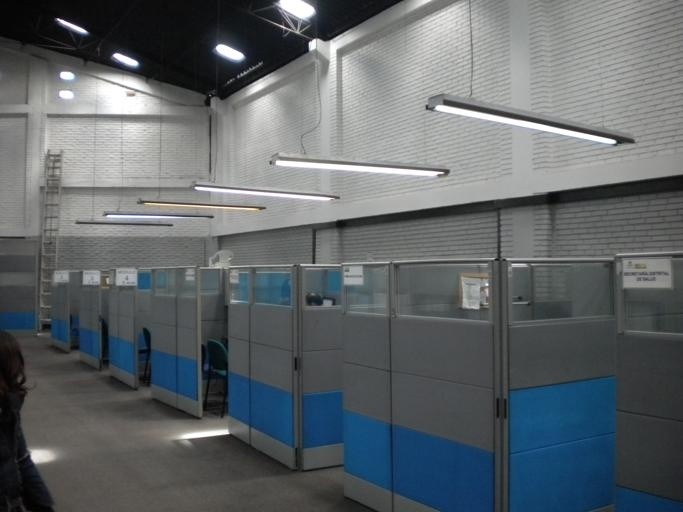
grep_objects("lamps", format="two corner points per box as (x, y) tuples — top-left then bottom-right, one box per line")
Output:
(74, 179), (341, 233)
(425, 92), (637, 153)
(268, 153), (451, 185)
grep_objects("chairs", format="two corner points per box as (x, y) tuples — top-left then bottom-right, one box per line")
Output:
(200, 338), (229, 418)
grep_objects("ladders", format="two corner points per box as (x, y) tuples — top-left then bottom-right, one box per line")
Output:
(38, 149), (65, 332)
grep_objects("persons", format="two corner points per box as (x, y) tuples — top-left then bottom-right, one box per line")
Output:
(0, 330), (57, 512)
